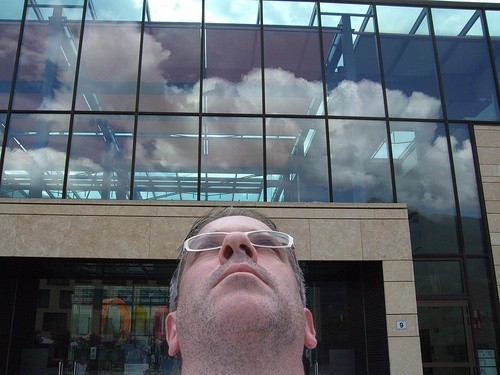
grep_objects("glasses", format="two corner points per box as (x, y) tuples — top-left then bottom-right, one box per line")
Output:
(178, 230), (298, 262)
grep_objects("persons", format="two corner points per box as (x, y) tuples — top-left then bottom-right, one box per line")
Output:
(165, 209), (317, 375)
